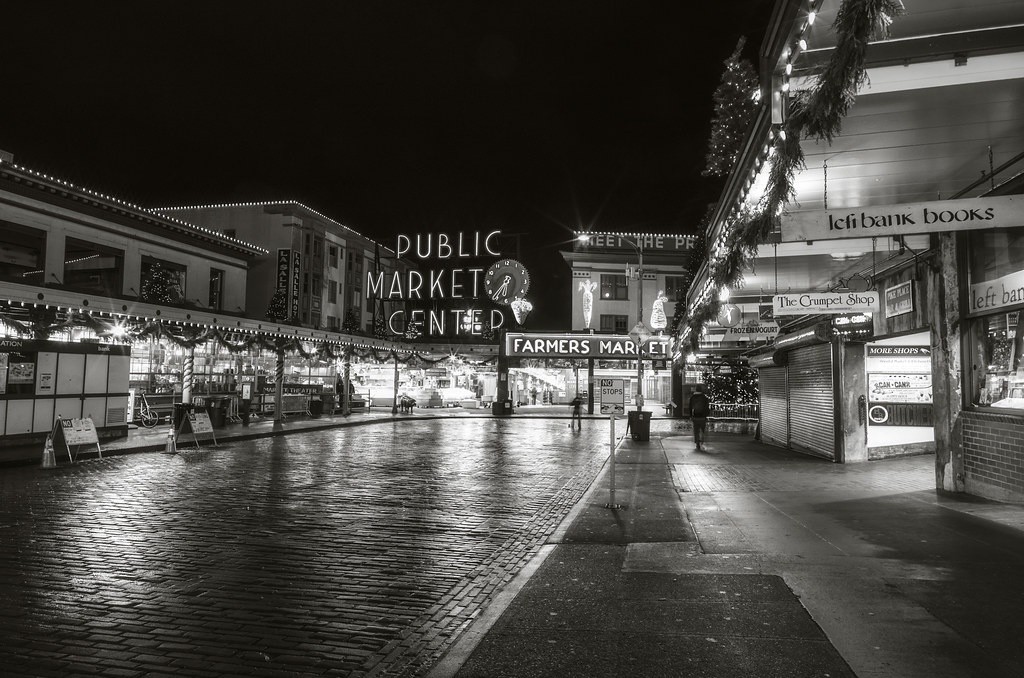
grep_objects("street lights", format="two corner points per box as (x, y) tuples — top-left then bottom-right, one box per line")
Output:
(579, 234), (644, 409)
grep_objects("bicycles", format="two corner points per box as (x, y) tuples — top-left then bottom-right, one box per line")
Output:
(133, 388), (159, 428)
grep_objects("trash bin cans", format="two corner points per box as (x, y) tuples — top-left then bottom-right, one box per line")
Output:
(174, 403), (195, 434)
(202, 396), (232, 429)
(502, 399), (512, 415)
(628, 411), (653, 443)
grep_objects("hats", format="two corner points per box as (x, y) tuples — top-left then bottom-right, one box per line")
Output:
(696, 385), (701, 391)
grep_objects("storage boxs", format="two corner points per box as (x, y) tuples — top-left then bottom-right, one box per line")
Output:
(461, 399), (481, 408)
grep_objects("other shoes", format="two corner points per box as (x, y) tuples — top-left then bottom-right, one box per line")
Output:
(696, 444), (700, 450)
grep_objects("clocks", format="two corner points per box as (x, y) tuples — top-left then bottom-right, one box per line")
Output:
(484, 259), (531, 305)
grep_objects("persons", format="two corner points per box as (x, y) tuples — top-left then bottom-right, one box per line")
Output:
(688, 383), (710, 450)
(569, 393), (582, 433)
(531, 387), (537, 405)
